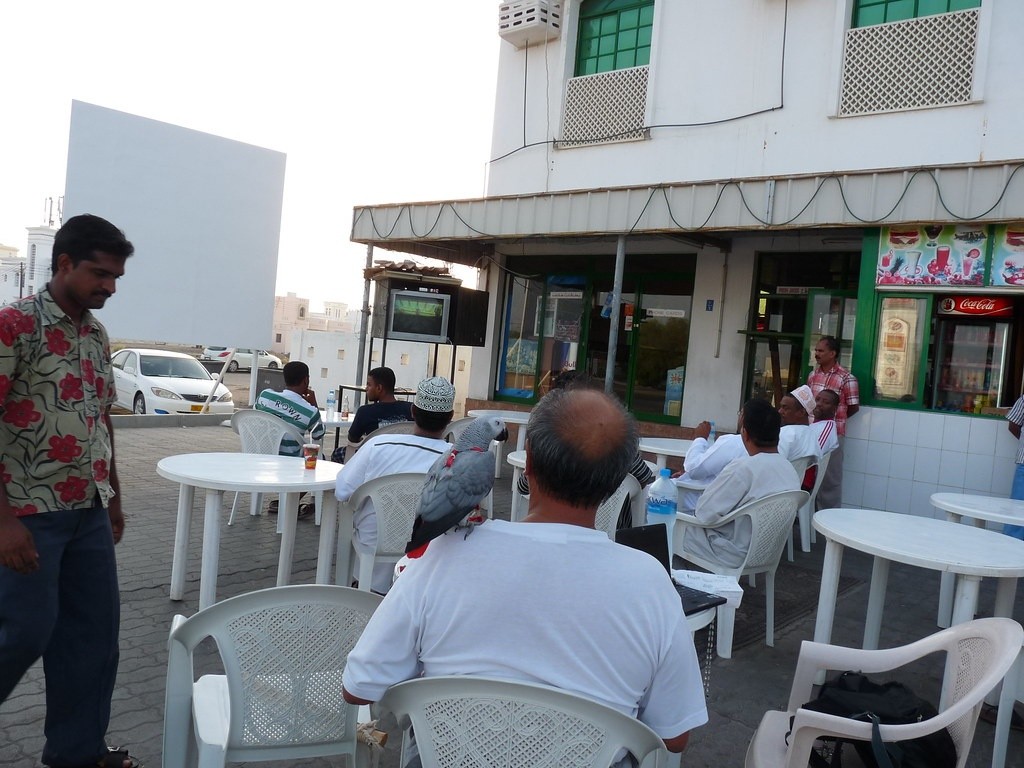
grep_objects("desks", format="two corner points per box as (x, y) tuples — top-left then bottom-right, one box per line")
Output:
(157, 454), (347, 612)
(812, 507), (1024, 705)
(507, 450), (660, 521)
(929, 493), (1024, 629)
(637, 437), (694, 469)
(468, 410), (531, 490)
(317, 411), (356, 459)
(393, 546), (717, 768)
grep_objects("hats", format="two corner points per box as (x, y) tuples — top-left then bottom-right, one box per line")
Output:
(414, 376), (456, 413)
(790, 385), (816, 415)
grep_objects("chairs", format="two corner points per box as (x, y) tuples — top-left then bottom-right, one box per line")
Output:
(162, 409), (1024, 768)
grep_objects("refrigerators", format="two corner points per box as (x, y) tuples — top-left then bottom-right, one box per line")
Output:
(932, 295), (1015, 417)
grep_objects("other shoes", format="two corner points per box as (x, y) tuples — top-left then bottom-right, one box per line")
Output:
(267, 500), (279, 513)
(299, 503), (315, 519)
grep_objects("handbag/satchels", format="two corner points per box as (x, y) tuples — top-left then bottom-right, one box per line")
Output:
(782, 669), (957, 768)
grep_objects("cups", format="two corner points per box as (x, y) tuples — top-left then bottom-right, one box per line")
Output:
(882, 256), (891, 267)
(906, 251), (921, 278)
(302, 443), (320, 470)
(961, 257), (974, 278)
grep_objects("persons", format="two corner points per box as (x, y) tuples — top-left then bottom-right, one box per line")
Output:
(801, 389), (840, 493)
(777, 384), (821, 490)
(670, 398), (772, 516)
(807, 335), (860, 524)
(671, 405), (801, 571)
(331, 368), (415, 464)
(1002, 395), (1024, 541)
(516, 370), (653, 532)
(334, 377), (460, 596)
(0, 214), (140, 768)
(253, 361), (326, 511)
(342, 382), (709, 768)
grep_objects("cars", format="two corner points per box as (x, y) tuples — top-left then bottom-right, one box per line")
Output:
(109, 347), (236, 416)
(200, 346), (284, 371)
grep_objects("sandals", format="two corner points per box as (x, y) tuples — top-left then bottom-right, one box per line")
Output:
(42, 745), (141, 768)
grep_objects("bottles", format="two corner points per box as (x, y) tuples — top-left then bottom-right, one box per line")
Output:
(705, 418), (716, 445)
(646, 468), (679, 576)
(936, 366), (997, 413)
(326, 389), (335, 419)
(341, 396), (350, 421)
(946, 324), (1003, 343)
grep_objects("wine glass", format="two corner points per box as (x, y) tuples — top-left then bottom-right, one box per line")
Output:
(925, 226), (943, 247)
(936, 246), (950, 278)
